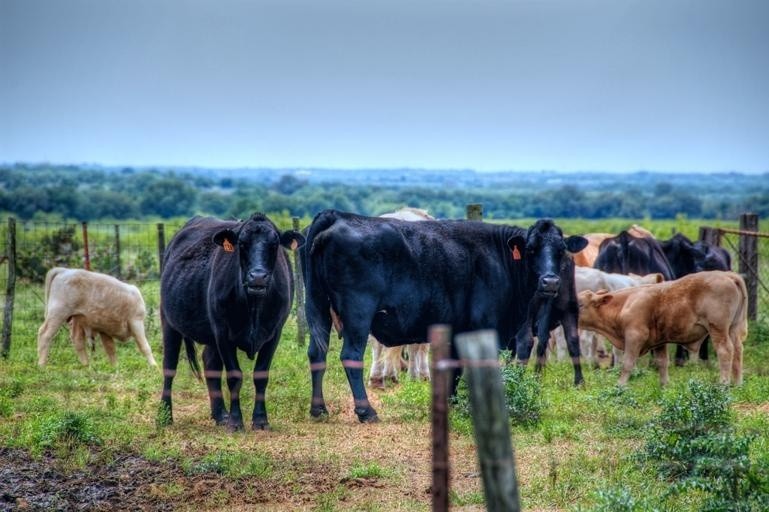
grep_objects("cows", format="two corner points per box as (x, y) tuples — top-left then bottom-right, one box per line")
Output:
(158, 210), (308, 433)
(296, 205), (749, 424)
(36, 266), (162, 368)
